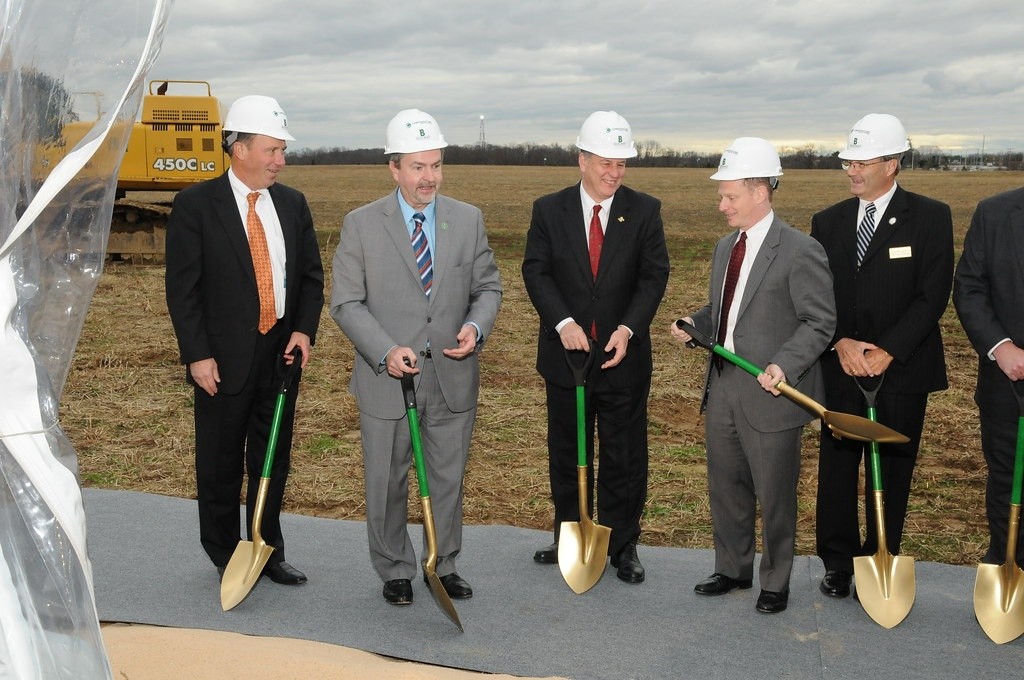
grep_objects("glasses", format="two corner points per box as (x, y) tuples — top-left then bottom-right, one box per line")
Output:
(840, 159), (888, 171)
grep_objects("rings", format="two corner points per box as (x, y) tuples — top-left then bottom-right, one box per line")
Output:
(850, 369), (856, 374)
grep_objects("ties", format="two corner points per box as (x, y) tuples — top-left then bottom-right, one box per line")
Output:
(714, 232), (748, 377)
(410, 212), (433, 299)
(246, 192), (278, 335)
(589, 206), (605, 342)
(856, 202), (876, 272)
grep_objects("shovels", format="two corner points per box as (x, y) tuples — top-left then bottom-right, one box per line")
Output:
(401, 357), (464, 633)
(220, 348), (301, 613)
(557, 337), (612, 595)
(973, 378), (1024, 644)
(851, 371), (917, 632)
(674, 318), (911, 443)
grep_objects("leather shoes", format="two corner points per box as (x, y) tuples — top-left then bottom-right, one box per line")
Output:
(694, 573), (752, 595)
(383, 579), (413, 604)
(819, 568), (860, 600)
(423, 572), (473, 599)
(610, 540), (645, 584)
(534, 541), (558, 564)
(755, 587), (790, 613)
(217, 560), (308, 585)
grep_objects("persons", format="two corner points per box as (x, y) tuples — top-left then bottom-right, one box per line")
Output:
(521, 111), (670, 584)
(165, 94), (325, 584)
(953, 186), (1023, 569)
(808, 115), (954, 602)
(329, 108), (501, 604)
(669, 136), (837, 614)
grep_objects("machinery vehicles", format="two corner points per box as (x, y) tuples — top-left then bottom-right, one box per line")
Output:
(0, 66), (231, 265)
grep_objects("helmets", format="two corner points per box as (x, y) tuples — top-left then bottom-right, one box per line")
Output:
(221, 95), (297, 141)
(838, 113), (911, 160)
(709, 137), (783, 181)
(383, 108), (449, 154)
(574, 110), (638, 158)
